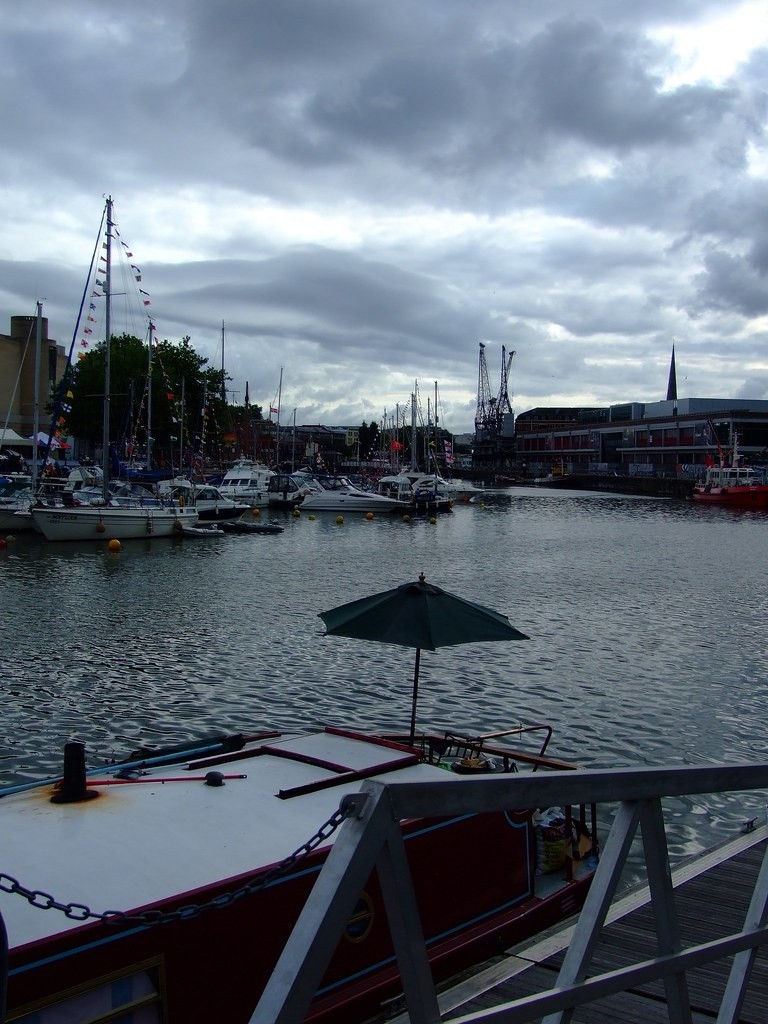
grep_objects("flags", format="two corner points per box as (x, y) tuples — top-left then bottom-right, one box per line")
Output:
(40, 217), (225, 480)
(444, 439), (452, 451)
(271, 408), (277, 413)
(446, 452), (456, 464)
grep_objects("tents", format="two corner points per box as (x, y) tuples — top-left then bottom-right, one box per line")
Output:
(0, 428), (34, 452)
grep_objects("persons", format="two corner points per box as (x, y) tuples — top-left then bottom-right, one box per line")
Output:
(178, 494), (186, 506)
(189, 472), (206, 485)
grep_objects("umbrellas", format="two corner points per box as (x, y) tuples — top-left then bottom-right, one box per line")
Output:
(317, 571), (531, 748)
(28, 431), (72, 450)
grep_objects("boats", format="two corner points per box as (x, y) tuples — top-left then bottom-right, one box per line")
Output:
(534, 456), (576, 488)
(0, 726), (600, 1024)
(693, 417), (768, 500)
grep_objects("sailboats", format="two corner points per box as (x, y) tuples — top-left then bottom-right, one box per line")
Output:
(0, 194), (485, 540)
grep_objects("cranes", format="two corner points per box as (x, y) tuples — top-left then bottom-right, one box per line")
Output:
(474, 342), (516, 481)
